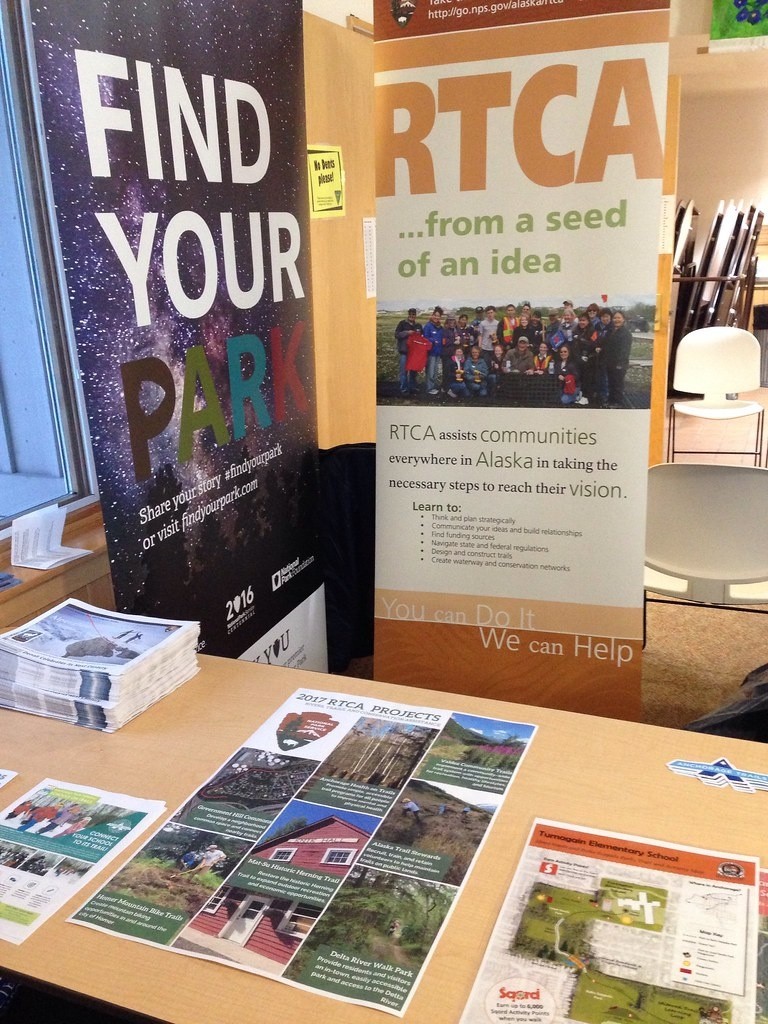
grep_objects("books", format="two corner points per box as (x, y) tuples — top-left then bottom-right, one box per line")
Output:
(0, 597), (201, 734)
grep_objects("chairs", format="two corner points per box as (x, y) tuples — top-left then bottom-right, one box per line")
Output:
(667, 326), (764, 466)
(642, 463), (768, 650)
(668, 199), (764, 399)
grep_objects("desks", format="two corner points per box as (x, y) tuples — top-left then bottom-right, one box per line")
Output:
(0, 625), (768, 1024)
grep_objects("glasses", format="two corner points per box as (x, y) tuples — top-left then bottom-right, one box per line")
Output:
(409, 314), (415, 315)
(588, 309), (596, 312)
(561, 350), (567, 353)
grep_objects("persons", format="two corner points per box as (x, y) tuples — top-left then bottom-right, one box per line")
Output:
(395, 308), (422, 393)
(0, 845), (48, 876)
(444, 301), (632, 405)
(5, 799), (92, 839)
(462, 802), (470, 817)
(401, 798), (420, 823)
(439, 800), (447, 815)
(180, 845), (227, 884)
(423, 306), (445, 394)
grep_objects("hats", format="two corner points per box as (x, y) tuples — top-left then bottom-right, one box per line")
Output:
(206, 845), (217, 850)
(549, 309), (559, 316)
(519, 336), (528, 342)
(475, 307), (484, 312)
(563, 299), (573, 306)
(408, 308), (416, 313)
(448, 315), (455, 319)
